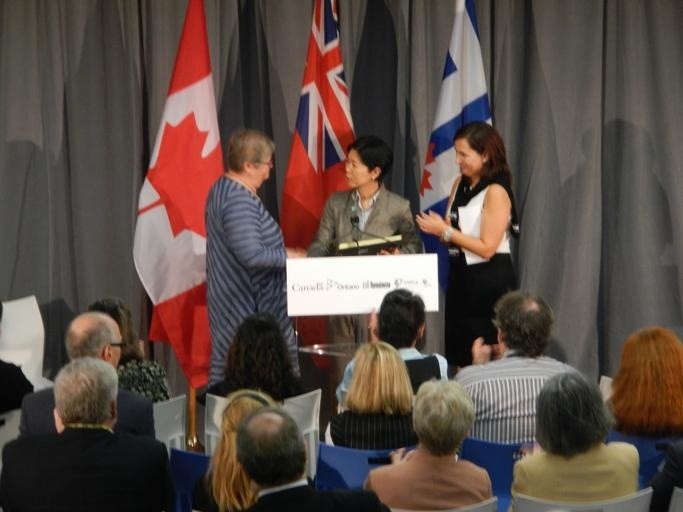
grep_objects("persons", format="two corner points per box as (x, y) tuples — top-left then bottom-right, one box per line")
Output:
(415, 121), (519, 366)
(607, 329), (683, 431)
(1, 301), (33, 414)
(0, 360), (177, 512)
(205, 130), (301, 391)
(236, 409), (389, 512)
(452, 294), (578, 444)
(365, 380), (492, 511)
(20, 313), (155, 437)
(336, 288), (449, 408)
(306, 135), (422, 255)
(192, 389), (274, 512)
(650, 441), (683, 512)
(514, 372), (639, 503)
(197, 313), (306, 406)
(325, 342), (413, 451)
(88, 296), (171, 403)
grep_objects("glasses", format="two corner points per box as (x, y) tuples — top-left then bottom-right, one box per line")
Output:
(254, 160), (275, 167)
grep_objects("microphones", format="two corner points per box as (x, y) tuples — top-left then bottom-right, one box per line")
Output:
(307, 216), (355, 256)
(355, 216), (412, 253)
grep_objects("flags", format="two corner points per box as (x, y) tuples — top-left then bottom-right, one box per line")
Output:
(134, 0), (226, 388)
(280, 1), (358, 372)
(419, 0), (494, 291)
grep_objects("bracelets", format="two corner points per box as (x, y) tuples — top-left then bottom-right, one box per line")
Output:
(443, 228), (453, 242)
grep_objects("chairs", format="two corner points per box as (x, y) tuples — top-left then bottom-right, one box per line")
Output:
(513, 487), (653, 512)
(153, 394), (187, 455)
(315, 442), (418, 491)
(392, 496), (498, 512)
(668, 487), (683, 512)
(203, 389), (321, 481)
(0, 409), (22, 469)
